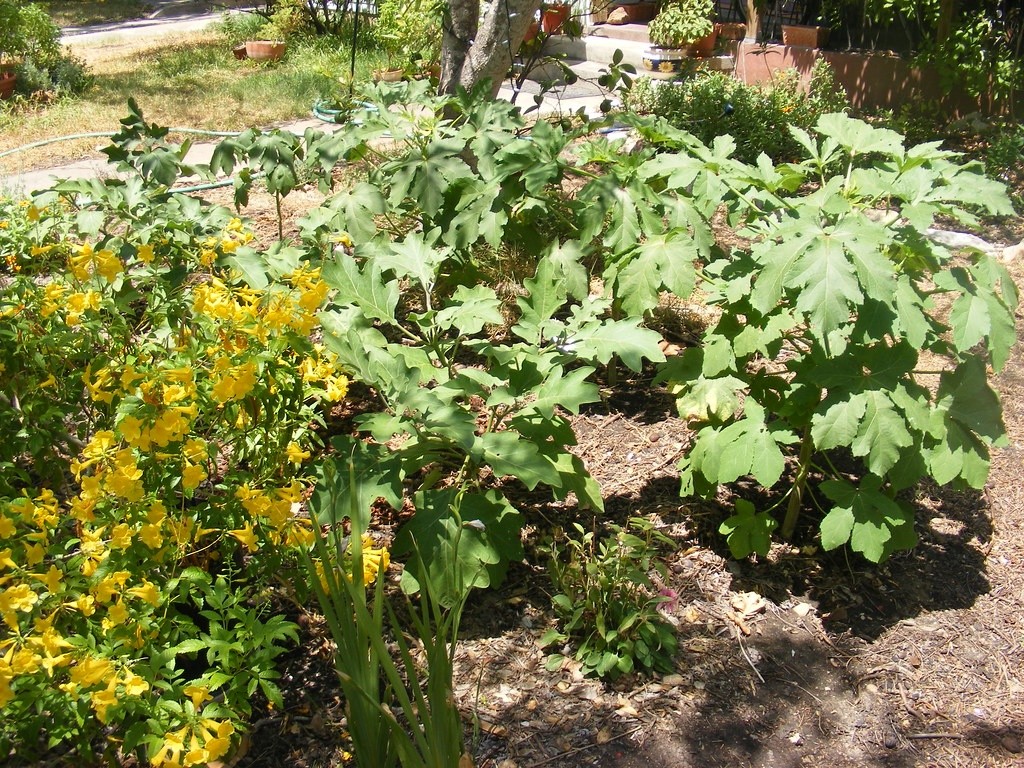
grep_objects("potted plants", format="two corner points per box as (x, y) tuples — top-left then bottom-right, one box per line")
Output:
(524, 16), (538, 44)
(372, 53), (404, 82)
(221, 9), (247, 60)
(245, 22), (289, 62)
(642, 1), (724, 80)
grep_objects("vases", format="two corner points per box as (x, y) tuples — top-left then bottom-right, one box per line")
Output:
(0, 72), (17, 99)
(781, 23), (832, 49)
(541, 3), (571, 35)
